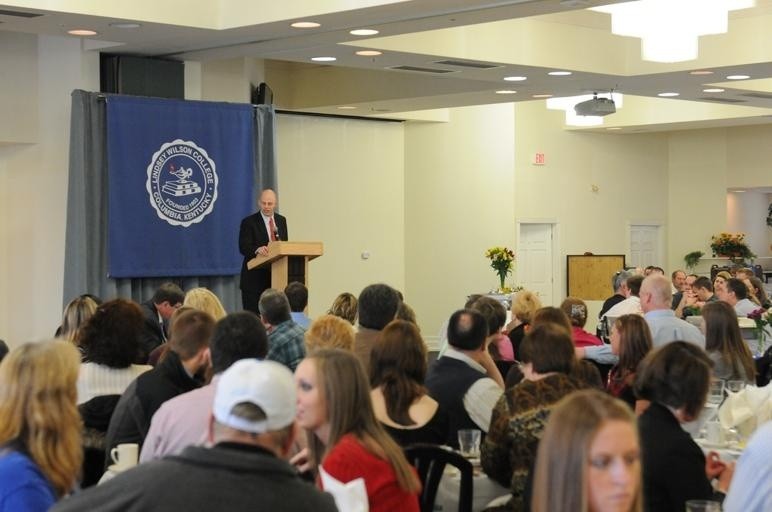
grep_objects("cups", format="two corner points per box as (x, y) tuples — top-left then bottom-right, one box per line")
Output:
(110, 442), (139, 470)
(706, 421), (727, 444)
(685, 498), (722, 512)
(706, 377), (745, 404)
(456, 428), (482, 462)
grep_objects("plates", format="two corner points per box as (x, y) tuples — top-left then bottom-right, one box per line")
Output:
(108, 464), (136, 475)
(696, 437), (732, 449)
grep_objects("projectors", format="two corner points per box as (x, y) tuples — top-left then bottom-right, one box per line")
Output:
(574, 98), (615, 117)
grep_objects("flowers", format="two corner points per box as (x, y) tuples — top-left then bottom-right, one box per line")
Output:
(710, 232), (758, 263)
(484, 246), (516, 288)
(747, 308), (765, 329)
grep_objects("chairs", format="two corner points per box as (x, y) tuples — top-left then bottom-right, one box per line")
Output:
(384, 439), (473, 512)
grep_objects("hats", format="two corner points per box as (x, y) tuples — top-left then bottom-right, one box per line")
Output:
(214, 358), (296, 433)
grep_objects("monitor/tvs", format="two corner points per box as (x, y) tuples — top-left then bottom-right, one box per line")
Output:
(258, 83), (274, 106)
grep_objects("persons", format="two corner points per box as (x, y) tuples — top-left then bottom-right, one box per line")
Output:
(238, 188), (289, 319)
(0, 264), (772, 512)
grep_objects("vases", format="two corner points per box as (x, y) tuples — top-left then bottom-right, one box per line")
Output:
(757, 329), (764, 345)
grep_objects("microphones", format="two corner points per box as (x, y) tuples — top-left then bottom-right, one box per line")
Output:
(274, 226), (281, 241)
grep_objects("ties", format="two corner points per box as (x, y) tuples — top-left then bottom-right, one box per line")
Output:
(269, 218), (277, 241)
